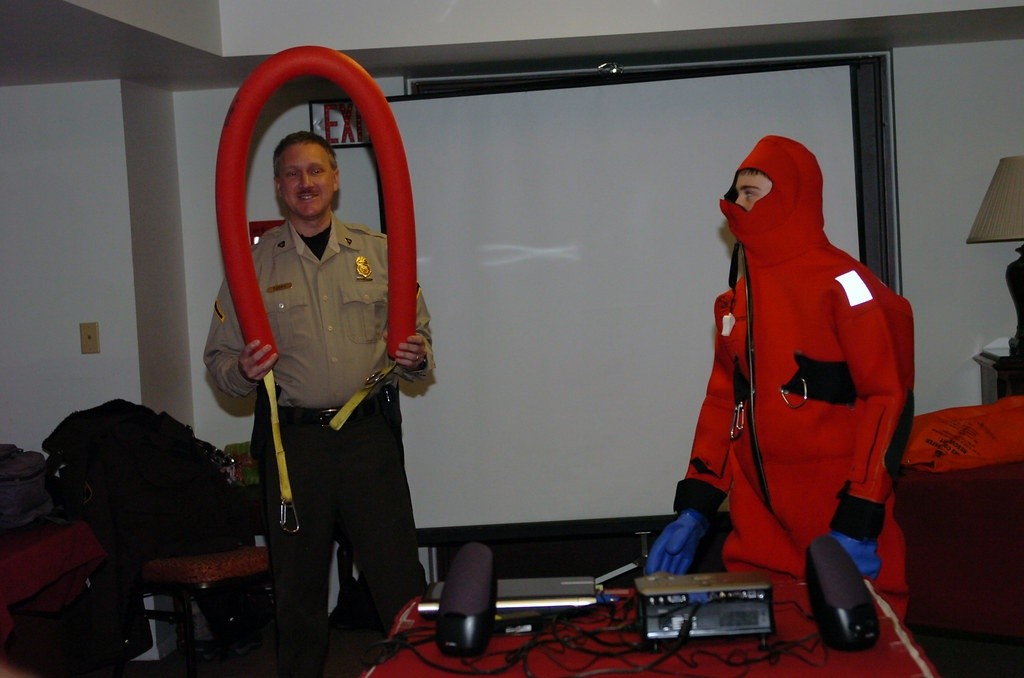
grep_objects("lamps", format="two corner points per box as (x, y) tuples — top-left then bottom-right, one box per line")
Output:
(966, 157), (1024, 354)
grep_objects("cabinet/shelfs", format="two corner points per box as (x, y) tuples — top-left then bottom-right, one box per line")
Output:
(970, 348), (1024, 407)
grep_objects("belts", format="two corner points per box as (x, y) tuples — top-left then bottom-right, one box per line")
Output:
(277, 397), (378, 425)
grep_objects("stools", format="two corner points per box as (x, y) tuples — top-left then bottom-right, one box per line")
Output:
(139, 545), (270, 678)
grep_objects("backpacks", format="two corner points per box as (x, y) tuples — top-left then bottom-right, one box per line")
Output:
(41, 399), (247, 564)
(0, 441), (53, 528)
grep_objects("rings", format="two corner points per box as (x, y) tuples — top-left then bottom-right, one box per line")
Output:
(416, 356), (419, 361)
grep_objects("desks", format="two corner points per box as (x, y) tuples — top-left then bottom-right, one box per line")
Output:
(353, 584), (939, 678)
(0, 516), (109, 660)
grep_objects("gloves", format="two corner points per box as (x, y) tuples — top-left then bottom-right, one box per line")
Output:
(830, 529), (882, 581)
(644, 508), (709, 578)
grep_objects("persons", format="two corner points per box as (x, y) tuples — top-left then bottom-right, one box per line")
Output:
(204, 131), (435, 628)
(645, 134), (914, 623)
